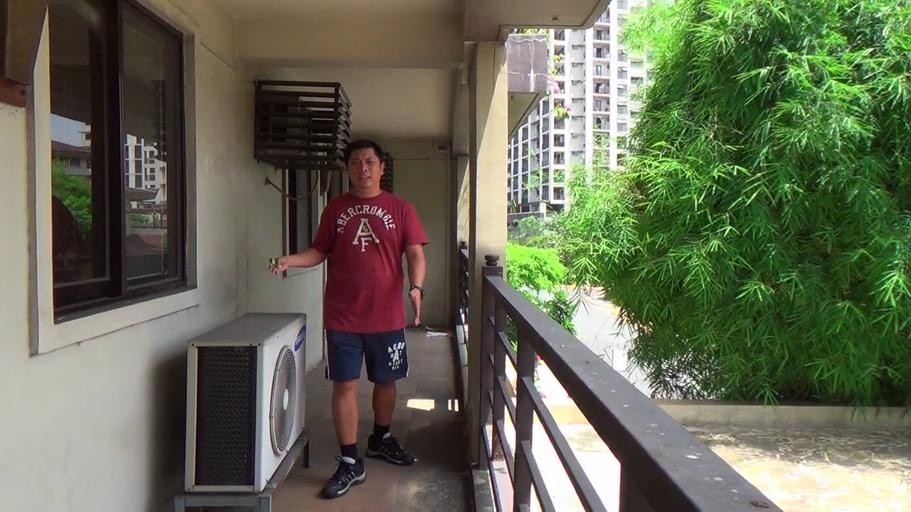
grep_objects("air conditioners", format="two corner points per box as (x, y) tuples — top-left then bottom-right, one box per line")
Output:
(185, 312), (308, 494)
(269, 89), (316, 159)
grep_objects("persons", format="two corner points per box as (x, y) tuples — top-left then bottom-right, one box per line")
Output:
(267, 140), (429, 499)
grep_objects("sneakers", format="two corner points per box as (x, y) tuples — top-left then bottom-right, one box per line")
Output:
(321, 457), (366, 498)
(367, 431), (419, 465)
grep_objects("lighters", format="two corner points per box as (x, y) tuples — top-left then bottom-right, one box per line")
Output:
(269, 257), (282, 269)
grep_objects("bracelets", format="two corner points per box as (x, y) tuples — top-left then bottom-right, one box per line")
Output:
(408, 285), (424, 301)
